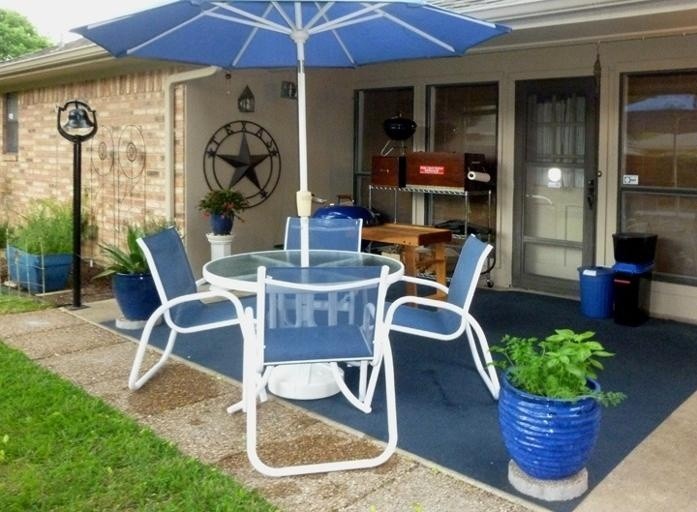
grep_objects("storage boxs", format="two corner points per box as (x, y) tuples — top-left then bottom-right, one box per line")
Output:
(371, 155), (407, 188)
(407, 150), (487, 192)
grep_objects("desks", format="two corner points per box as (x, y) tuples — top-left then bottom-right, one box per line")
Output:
(357, 220), (452, 306)
(200, 249), (406, 413)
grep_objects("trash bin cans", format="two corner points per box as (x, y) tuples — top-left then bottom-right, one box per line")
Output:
(577, 265), (615, 320)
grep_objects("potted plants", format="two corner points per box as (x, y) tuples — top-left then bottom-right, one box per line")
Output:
(476, 326), (629, 505)
(95, 220), (160, 330)
(5, 195), (91, 293)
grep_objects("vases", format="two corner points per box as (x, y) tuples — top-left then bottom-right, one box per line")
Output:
(209, 210), (233, 235)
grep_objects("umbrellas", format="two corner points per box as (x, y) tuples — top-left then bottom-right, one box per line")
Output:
(66, 1), (514, 271)
(622, 91), (696, 190)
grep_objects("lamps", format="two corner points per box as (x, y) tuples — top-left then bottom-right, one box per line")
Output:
(54, 96), (99, 310)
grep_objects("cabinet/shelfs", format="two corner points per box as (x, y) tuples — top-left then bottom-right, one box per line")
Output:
(364, 183), (495, 291)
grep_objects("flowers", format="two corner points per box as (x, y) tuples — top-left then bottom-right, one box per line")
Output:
(196, 186), (249, 224)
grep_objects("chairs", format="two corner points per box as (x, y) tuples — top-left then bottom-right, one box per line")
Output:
(228, 265), (399, 474)
(126, 226), (259, 397)
(359, 229), (503, 407)
(279, 213), (363, 254)
